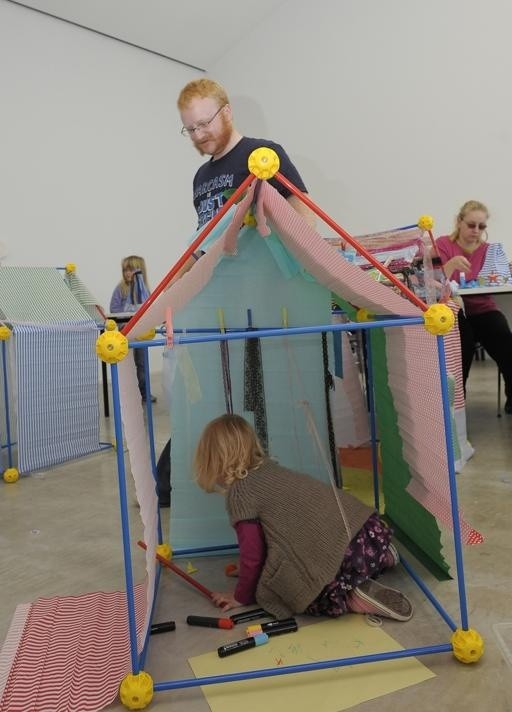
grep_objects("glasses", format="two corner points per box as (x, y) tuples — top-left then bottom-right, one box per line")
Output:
(463, 219), (487, 230)
(182, 106), (223, 137)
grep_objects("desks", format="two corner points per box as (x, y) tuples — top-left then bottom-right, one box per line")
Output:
(436, 273), (511, 299)
(96, 311), (136, 417)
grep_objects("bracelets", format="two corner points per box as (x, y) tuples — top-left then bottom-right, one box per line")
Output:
(192, 253), (199, 260)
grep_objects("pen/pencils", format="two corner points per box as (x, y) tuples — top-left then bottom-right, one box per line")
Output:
(149, 621), (176, 634)
(230, 608), (270, 621)
(187, 615), (233, 628)
(246, 617), (298, 636)
(218, 632), (269, 658)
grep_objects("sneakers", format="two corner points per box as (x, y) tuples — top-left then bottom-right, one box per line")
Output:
(384, 544), (400, 570)
(346, 577), (413, 625)
(142, 394), (154, 403)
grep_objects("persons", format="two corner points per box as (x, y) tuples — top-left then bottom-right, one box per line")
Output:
(429, 200), (510, 415)
(164, 78), (316, 292)
(109, 255), (159, 404)
(191, 412), (414, 621)
